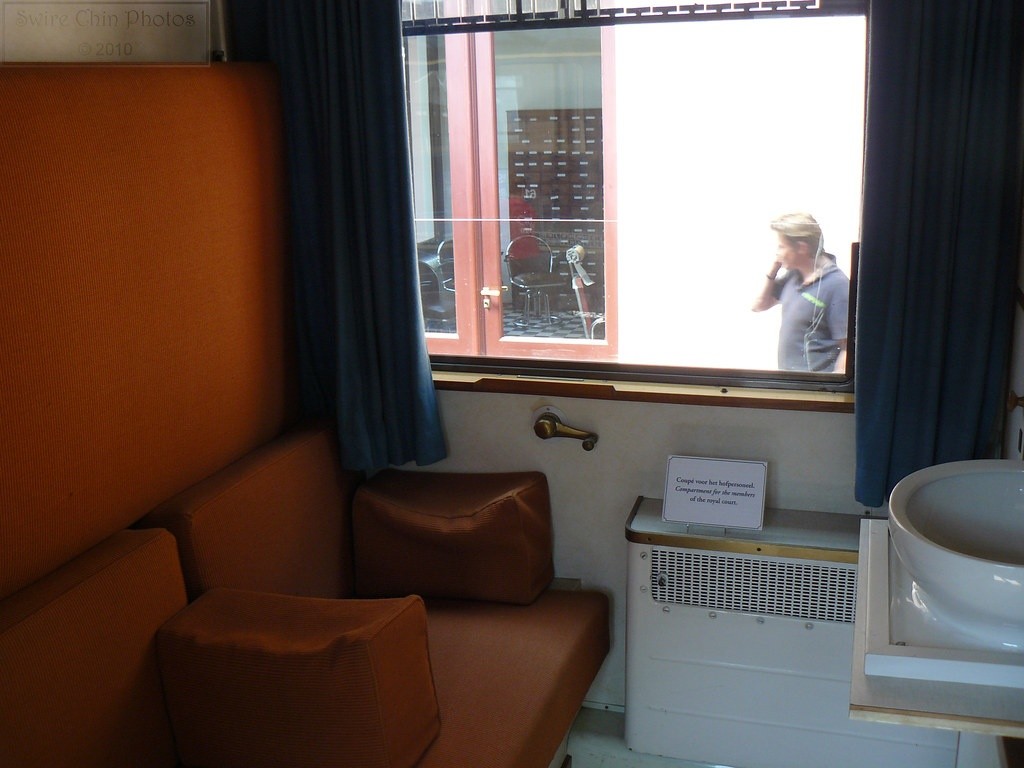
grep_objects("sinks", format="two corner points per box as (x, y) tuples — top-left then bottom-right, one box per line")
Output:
(887, 458), (1024, 655)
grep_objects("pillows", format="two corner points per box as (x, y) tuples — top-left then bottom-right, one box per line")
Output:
(155, 586), (441, 768)
(353, 468), (555, 604)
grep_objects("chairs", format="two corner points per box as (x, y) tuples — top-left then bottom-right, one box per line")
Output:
(505, 235), (568, 326)
(417, 239), (457, 333)
(566, 245), (606, 340)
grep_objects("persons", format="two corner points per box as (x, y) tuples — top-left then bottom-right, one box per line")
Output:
(751, 212), (850, 372)
(509, 176), (538, 306)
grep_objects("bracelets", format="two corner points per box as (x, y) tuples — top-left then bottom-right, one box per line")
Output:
(766, 273), (777, 279)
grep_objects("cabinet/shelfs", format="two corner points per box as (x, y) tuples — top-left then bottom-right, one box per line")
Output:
(505, 109), (606, 314)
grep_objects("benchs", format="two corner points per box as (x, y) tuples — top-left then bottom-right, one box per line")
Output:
(0, 428), (613, 767)
